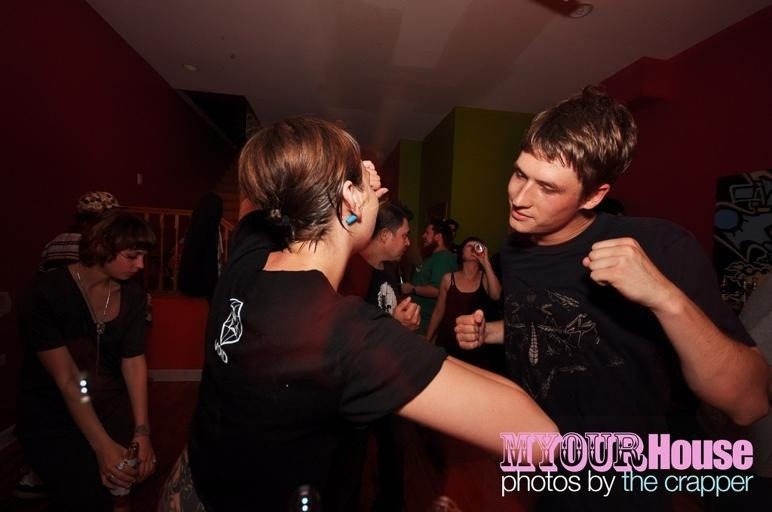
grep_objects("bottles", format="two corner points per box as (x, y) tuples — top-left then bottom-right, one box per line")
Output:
(109, 440), (140, 498)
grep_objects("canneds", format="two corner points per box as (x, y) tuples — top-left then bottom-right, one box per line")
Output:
(471, 243), (484, 256)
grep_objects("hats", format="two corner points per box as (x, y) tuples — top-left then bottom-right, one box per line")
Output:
(77, 191), (129, 213)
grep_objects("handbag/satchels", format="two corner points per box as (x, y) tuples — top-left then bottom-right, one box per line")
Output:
(162, 444), (204, 512)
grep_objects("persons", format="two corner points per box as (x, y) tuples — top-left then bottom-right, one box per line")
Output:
(16, 94), (772, 512)
